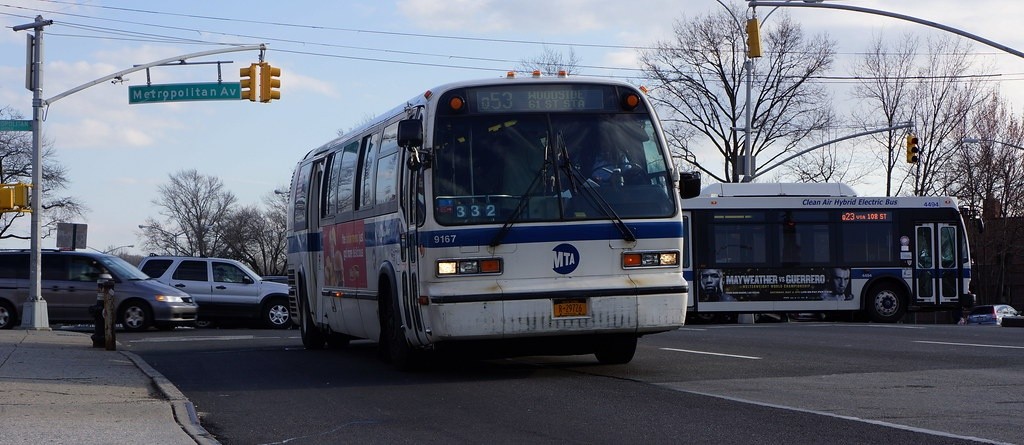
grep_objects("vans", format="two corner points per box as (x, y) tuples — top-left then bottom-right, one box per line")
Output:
(0, 248), (199, 333)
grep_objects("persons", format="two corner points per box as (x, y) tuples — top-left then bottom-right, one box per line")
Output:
(216, 267), (233, 282)
(305, 137), (652, 211)
(698, 269), (739, 303)
(821, 266), (852, 301)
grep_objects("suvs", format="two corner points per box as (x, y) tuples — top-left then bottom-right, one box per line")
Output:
(137, 253), (289, 330)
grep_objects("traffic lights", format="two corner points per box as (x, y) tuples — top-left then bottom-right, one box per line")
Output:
(907, 136), (918, 163)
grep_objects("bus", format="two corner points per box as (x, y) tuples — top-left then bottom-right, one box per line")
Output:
(286, 70), (701, 369)
(681, 183), (976, 323)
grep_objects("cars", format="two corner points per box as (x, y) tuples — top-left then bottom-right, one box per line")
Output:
(966, 304), (1023, 327)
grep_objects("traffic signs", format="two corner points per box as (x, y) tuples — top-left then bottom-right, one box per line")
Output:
(128, 82), (242, 105)
(0, 120), (33, 131)
